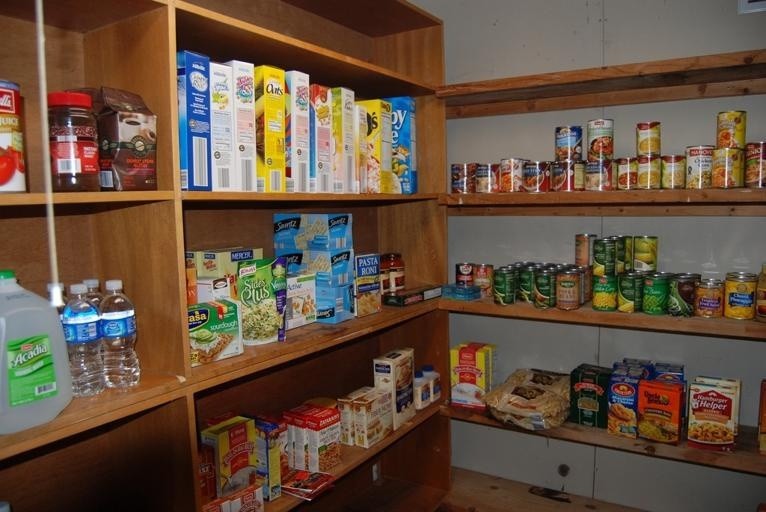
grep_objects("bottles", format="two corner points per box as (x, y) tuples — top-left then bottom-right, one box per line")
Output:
(98, 279), (141, 388)
(47, 282), (70, 324)
(756, 263), (766, 324)
(61, 284), (106, 397)
(83, 279), (104, 308)
(380, 252), (406, 303)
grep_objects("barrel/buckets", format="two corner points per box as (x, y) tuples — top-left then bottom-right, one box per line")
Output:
(0, 270), (73, 434)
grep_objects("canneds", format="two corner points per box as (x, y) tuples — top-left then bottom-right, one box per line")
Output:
(554, 119), (661, 192)
(662, 111), (766, 188)
(455, 263), (493, 297)
(642, 272), (757, 321)
(0, 79), (27, 193)
(556, 234), (657, 312)
(380, 254), (406, 298)
(451, 159), (552, 194)
(492, 261), (574, 309)
